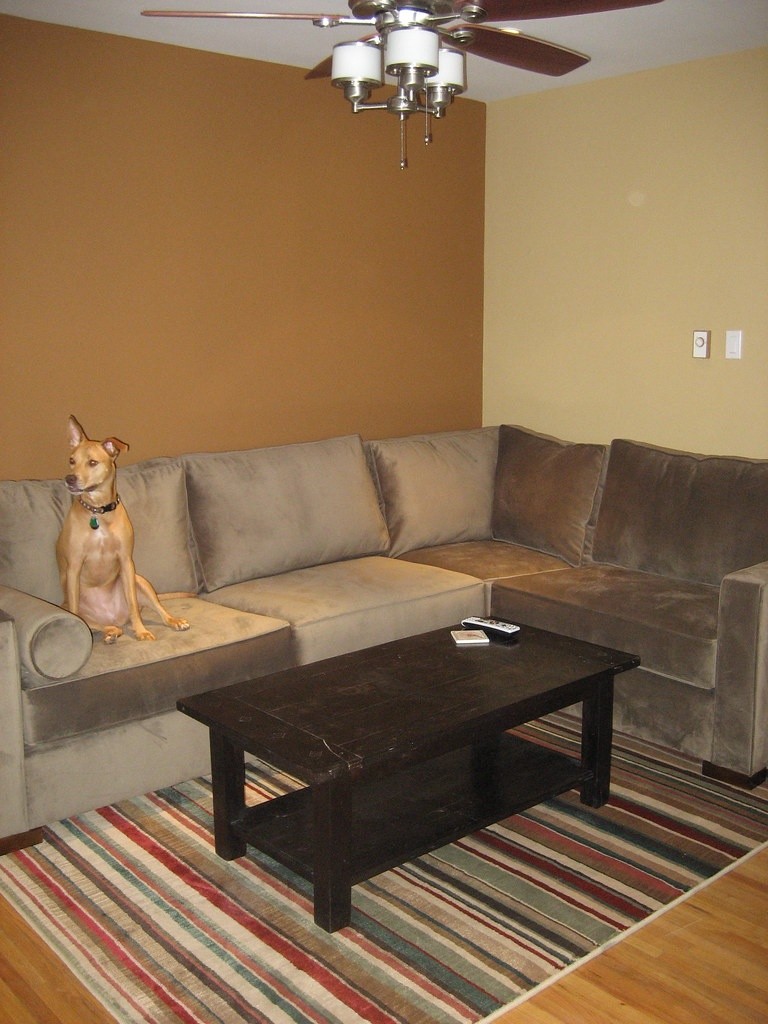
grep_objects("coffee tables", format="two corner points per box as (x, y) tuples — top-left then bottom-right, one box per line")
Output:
(174, 616), (639, 931)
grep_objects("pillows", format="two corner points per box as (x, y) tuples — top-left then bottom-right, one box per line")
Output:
(186, 434), (389, 591)
(489, 426), (605, 568)
(368, 426), (504, 558)
(595, 440), (768, 586)
(0, 457), (194, 610)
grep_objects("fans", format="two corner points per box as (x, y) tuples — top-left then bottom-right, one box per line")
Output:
(144, 0), (666, 76)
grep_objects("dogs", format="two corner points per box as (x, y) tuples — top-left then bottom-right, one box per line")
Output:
(55, 414), (190, 645)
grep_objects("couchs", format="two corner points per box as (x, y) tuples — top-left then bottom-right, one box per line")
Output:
(0, 425), (768, 846)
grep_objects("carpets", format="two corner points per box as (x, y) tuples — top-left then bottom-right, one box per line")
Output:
(0, 712), (768, 1024)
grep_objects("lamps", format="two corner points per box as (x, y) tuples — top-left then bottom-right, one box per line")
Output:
(331, 28), (464, 171)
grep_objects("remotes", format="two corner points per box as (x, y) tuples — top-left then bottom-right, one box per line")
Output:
(460, 616), (520, 638)
